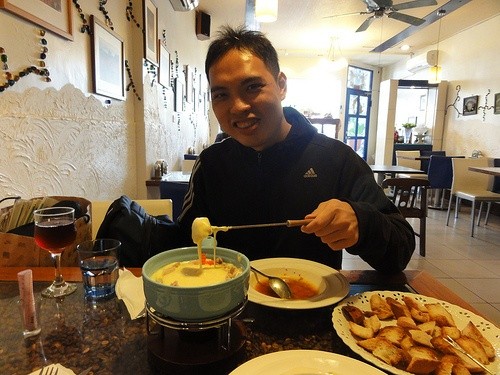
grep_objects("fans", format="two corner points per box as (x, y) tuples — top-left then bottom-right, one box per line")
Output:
(322, 0), (438, 34)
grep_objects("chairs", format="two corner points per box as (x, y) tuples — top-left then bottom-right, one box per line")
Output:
(159, 180), (191, 224)
(89, 199), (173, 245)
(381, 150), (500, 258)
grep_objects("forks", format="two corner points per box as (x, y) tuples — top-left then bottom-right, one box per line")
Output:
(247, 263), (293, 299)
(39, 365), (59, 375)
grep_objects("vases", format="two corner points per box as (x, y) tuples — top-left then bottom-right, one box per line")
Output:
(404, 128), (411, 144)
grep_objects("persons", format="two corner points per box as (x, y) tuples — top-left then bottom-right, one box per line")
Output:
(175, 26), (416, 273)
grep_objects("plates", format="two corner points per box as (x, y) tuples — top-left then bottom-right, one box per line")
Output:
(330, 287), (500, 375)
(240, 257), (350, 309)
(228, 348), (388, 375)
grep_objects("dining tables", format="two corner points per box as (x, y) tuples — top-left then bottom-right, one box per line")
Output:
(0, 265), (488, 375)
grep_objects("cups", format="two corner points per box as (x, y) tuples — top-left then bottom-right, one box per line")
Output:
(77, 239), (121, 298)
(33, 206), (77, 297)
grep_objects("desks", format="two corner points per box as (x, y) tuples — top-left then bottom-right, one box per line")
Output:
(394, 154), (465, 212)
(370, 164), (426, 205)
(145, 171), (194, 187)
(467, 166), (500, 177)
(310, 117), (340, 140)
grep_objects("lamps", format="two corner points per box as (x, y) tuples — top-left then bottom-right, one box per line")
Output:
(253, 0), (278, 22)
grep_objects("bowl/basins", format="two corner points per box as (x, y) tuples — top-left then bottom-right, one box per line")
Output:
(141, 247), (250, 321)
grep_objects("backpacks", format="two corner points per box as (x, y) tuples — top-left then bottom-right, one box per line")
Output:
(6, 200), (90, 238)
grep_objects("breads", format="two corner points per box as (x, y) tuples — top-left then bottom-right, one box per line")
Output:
(343, 294), (496, 375)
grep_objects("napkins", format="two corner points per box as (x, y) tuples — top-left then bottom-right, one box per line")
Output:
(114, 267), (148, 321)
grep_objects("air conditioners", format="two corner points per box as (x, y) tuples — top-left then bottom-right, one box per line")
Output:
(169, 0), (199, 13)
(407, 49), (439, 74)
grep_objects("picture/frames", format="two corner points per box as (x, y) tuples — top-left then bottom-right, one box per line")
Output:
(494, 93), (500, 114)
(142, 0), (160, 68)
(175, 77), (184, 113)
(90, 15), (127, 102)
(463, 96), (478, 116)
(186, 64), (210, 116)
(158, 39), (172, 90)
(0, 0), (75, 42)
